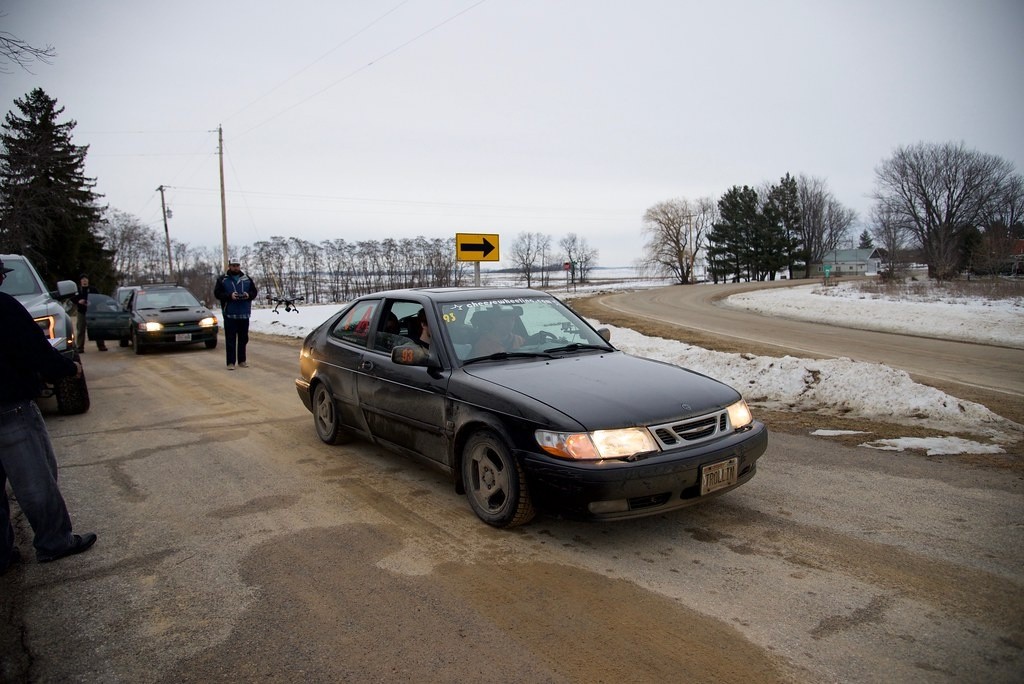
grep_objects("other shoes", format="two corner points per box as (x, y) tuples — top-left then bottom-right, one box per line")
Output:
(37, 532), (96, 561)
(99, 344), (107, 350)
(227, 363), (235, 370)
(239, 361), (249, 367)
(76, 348), (85, 353)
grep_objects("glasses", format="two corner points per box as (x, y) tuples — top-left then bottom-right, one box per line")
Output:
(230, 265), (240, 267)
(0, 273), (7, 279)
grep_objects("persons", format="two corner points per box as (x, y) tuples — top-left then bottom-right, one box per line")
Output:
(71, 274), (108, 353)
(404, 307), (432, 355)
(472, 311), (526, 357)
(0, 259), (98, 576)
(214, 257), (257, 370)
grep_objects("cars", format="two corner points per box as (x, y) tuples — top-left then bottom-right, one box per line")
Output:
(83, 284), (218, 356)
(292, 286), (768, 531)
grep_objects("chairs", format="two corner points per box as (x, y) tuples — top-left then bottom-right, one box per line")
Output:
(469, 310), (486, 347)
(405, 319), (423, 340)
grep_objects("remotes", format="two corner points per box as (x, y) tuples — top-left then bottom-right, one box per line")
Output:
(235, 292), (248, 300)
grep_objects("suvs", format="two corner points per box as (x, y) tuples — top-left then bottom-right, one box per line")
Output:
(1, 253), (91, 417)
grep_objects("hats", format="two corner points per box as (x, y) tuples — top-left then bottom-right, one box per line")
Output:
(80, 273), (89, 279)
(228, 258), (240, 265)
(0, 259), (15, 275)
